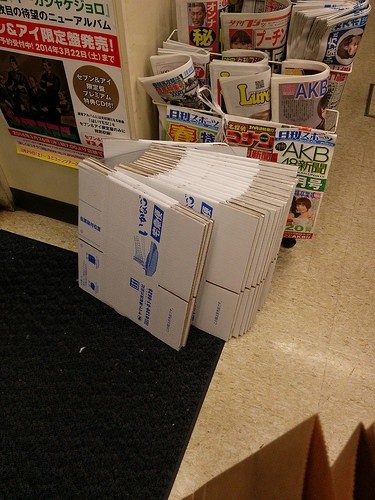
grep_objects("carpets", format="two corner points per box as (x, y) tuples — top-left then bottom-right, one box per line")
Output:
(0, 227), (227, 499)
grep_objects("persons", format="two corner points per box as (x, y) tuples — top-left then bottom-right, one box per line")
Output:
(287, 195), (300, 224)
(0, 1), (372, 145)
(292, 197), (314, 230)
